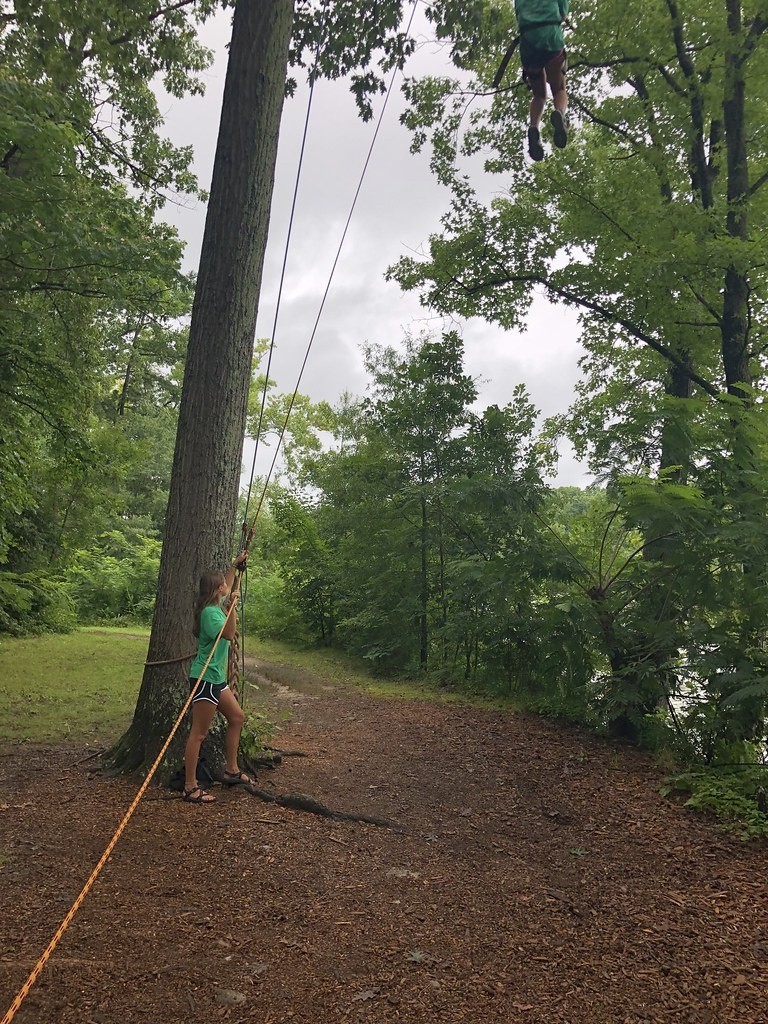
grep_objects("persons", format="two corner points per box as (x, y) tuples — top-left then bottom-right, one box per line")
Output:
(515, 0), (569, 161)
(181, 549), (256, 802)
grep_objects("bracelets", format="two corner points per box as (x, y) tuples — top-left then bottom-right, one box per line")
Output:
(232, 563), (237, 567)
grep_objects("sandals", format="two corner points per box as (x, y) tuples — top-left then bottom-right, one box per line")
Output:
(223, 770), (256, 786)
(182, 787), (218, 802)
